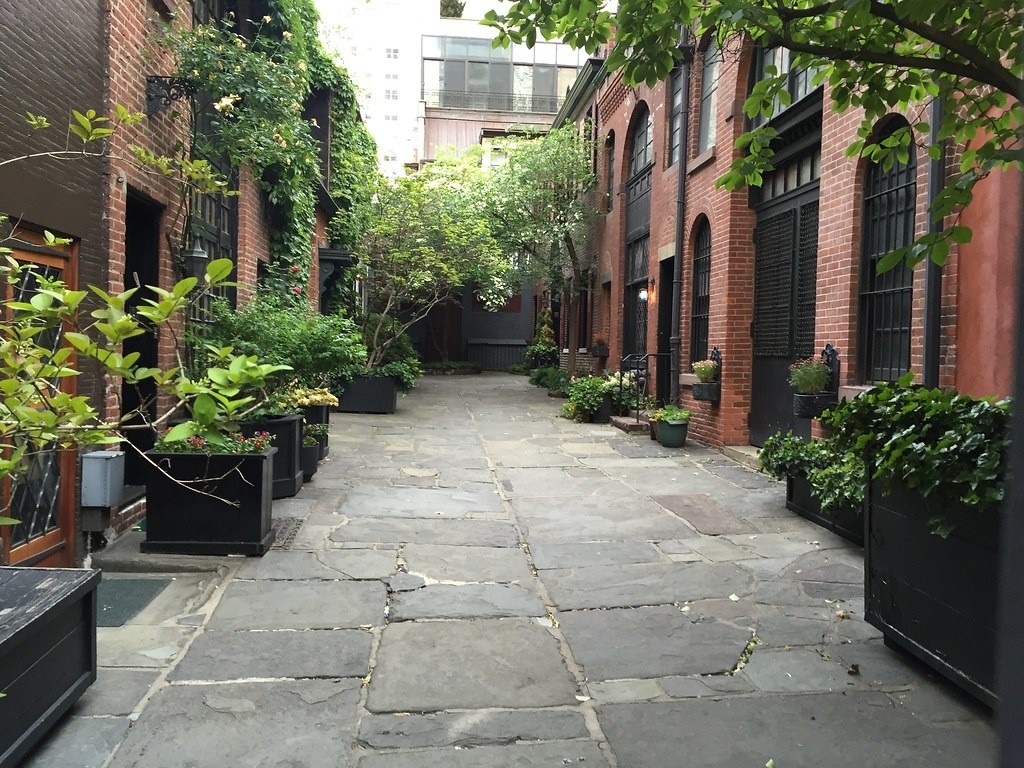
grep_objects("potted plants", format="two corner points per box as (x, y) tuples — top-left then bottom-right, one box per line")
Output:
(217, 241), (462, 499)
(571, 377), (615, 424)
(760, 371), (1009, 701)
(647, 405), (691, 448)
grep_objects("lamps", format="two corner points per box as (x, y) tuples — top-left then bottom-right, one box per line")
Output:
(179, 233), (211, 292)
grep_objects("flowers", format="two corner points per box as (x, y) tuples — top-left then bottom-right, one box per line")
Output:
(785, 356), (832, 396)
(151, 426), (270, 452)
(691, 360), (718, 385)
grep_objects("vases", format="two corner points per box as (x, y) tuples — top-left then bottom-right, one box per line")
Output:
(693, 382), (719, 401)
(793, 388), (836, 418)
(143, 445), (278, 557)
